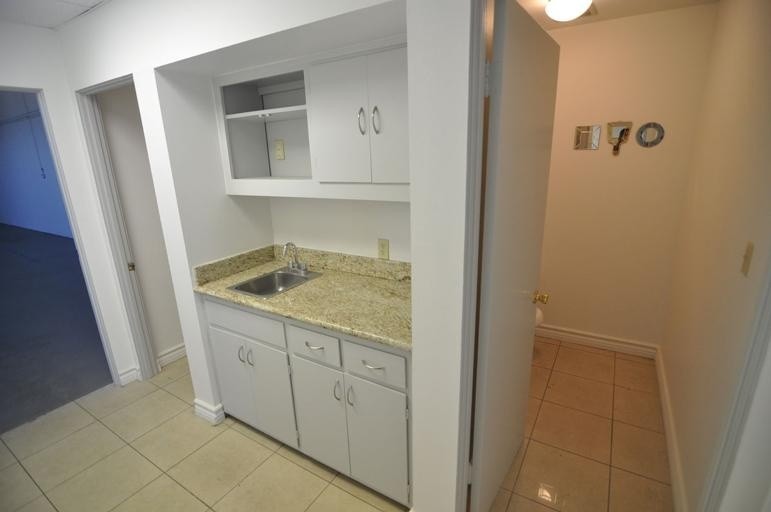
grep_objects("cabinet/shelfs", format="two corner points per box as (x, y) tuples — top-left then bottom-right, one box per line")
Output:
(286, 316), (411, 512)
(304, 34), (411, 203)
(199, 293), (296, 452)
(217, 51), (310, 200)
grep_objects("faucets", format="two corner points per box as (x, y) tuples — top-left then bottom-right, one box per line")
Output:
(282, 242), (308, 276)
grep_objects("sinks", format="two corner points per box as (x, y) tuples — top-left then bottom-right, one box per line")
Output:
(225, 266), (323, 300)
